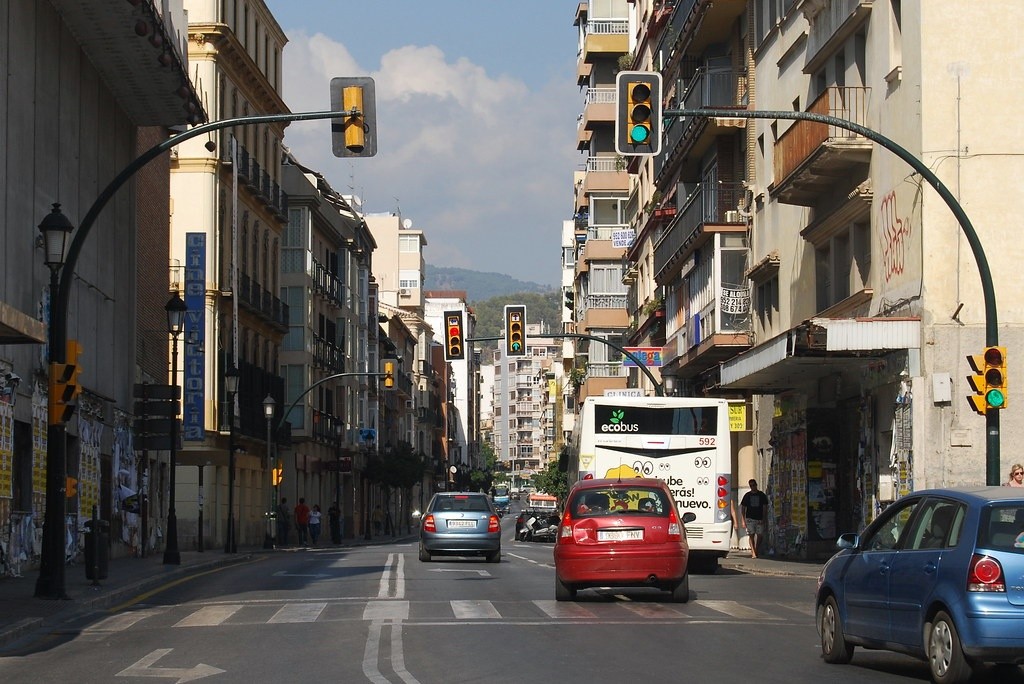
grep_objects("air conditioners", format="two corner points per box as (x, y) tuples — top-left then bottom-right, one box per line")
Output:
(726, 209), (737, 222)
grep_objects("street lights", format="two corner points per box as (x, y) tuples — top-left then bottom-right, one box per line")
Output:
(263, 392), (275, 550)
(162, 291), (187, 567)
(34, 203), (79, 600)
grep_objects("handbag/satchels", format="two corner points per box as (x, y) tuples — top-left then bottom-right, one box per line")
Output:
(285, 519), (291, 529)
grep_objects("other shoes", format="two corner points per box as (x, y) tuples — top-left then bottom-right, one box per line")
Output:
(756, 554), (759, 558)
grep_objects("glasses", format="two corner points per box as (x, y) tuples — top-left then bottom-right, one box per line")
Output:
(750, 484), (756, 487)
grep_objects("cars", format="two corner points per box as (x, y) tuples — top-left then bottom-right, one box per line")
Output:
(815, 486), (1024, 684)
(511, 493), (520, 500)
(553, 478), (696, 603)
(491, 501), (511, 516)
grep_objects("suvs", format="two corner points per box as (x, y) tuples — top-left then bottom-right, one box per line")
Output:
(515, 512), (540, 540)
(411, 488), (502, 562)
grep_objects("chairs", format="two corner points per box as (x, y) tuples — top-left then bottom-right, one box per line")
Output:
(591, 493), (609, 509)
(638, 496), (656, 512)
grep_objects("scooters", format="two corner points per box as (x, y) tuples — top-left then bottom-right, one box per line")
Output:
(520, 509), (558, 542)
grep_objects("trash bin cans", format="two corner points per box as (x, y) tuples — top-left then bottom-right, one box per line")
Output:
(265, 512), (278, 538)
(83, 505), (110, 587)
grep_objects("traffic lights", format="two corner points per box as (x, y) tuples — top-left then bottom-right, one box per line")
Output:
(984, 345), (1007, 410)
(272, 468), (283, 485)
(48, 339), (83, 424)
(615, 71), (663, 156)
(442, 310), (466, 362)
(504, 305), (527, 357)
(965, 354), (986, 416)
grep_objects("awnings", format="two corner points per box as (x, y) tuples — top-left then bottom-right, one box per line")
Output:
(720, 317), (921, 390)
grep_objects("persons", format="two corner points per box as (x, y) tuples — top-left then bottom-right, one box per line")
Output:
(372, 505), (383, 536)
(276, 497), (290, 546)
(309, 505), (322, 549)
(601, 494), (610, 510)
(740, 479), (768, 559)
(295, 498), (310, 547)
(1002, 464), (1024, 488)
(326, 502), (341, 545)
(721, 499), (738, 559)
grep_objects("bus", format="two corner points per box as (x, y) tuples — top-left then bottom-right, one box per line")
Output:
(494, 484), (509, 503)
(559, 396), (732, 573)
